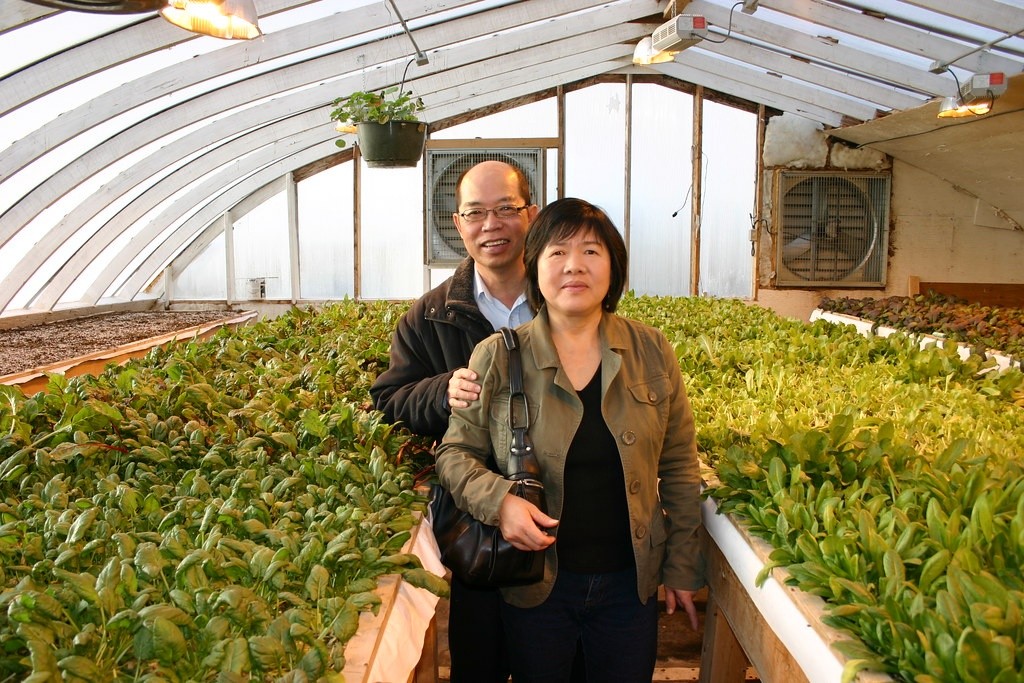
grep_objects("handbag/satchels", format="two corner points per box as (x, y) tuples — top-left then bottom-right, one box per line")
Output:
(430, 327), (546, 590)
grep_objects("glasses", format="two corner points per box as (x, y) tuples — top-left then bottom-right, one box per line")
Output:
(455, 204), (528, 222)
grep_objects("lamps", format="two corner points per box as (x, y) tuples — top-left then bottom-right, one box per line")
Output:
(936, 96), (991, 119)
(633, 37), (675, 66)
(157, 0), (262, 41)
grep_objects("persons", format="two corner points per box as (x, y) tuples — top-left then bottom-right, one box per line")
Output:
(434, 198), (708, 683)
(370, 160), (537, 683)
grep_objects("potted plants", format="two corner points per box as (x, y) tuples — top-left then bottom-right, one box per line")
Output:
(330, 86), (431, 168)
